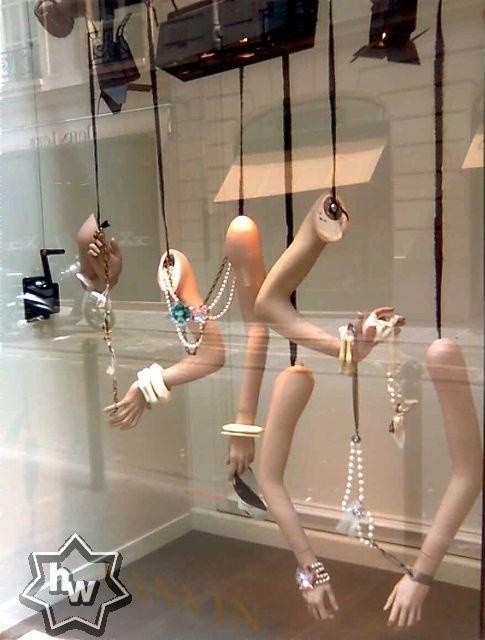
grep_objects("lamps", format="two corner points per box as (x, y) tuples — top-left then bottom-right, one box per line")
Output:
(82, 0), (140, 119)
(348, 1), (436, 66)
